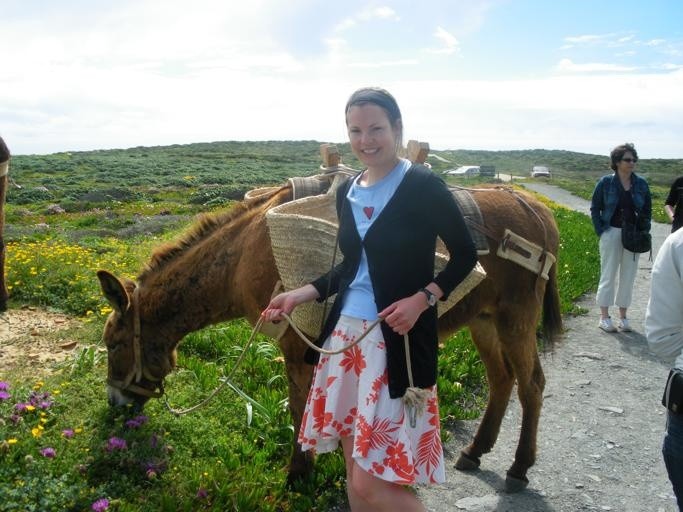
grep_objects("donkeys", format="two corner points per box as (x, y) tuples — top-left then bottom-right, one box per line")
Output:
(92, 173), (566, 496)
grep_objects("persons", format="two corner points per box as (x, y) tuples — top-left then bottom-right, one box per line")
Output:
(589, 142), (652, 333)
(644, 223), (683, 512)
(663, 176), (683, 233)
(262, 86), (478, 512)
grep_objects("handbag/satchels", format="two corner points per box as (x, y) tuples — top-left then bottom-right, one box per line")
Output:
(663, 368), (683, 417)
(619, 207), (653, 254)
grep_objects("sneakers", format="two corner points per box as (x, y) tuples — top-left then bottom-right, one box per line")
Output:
(597, 316), (632, 335)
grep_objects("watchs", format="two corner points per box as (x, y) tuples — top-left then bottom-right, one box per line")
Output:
(418, 288), (437, 306)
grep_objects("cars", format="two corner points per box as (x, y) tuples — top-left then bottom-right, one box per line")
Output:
(443, 164), (553, 184)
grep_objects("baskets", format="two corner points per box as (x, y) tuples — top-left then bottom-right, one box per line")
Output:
(263, 173), (487, 341)
